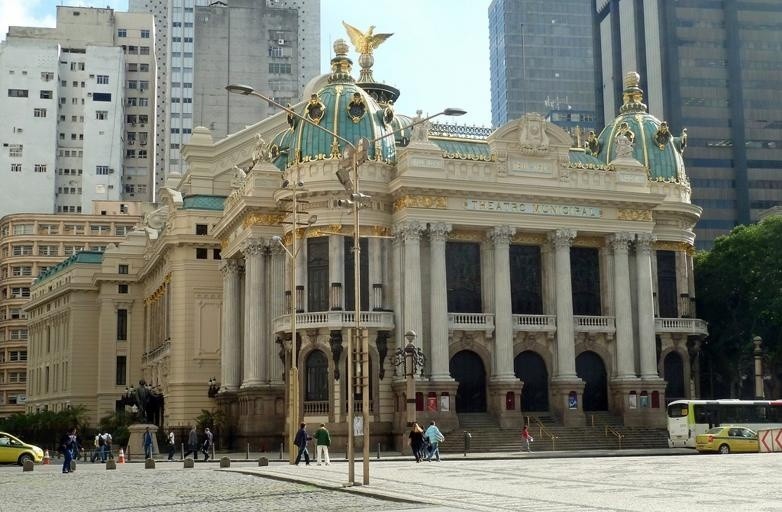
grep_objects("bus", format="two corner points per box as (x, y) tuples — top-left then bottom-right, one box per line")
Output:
(666, 399), (782, 448)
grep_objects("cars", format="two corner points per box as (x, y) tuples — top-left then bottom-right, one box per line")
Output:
(696, 427), (758, 454)
(0, 431), (44, 466)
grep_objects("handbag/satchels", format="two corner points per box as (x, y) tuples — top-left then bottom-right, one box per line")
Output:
(59, 443), (67, 453)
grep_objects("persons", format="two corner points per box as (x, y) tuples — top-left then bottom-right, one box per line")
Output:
(136, 380), (150, 423)
(617, 130), (634, 156)
(254, 134), (264, 159)
(409, 110), (431, 142)
(408, 421), (445, 463)
(294, 422), (312, 465)
(62, 428), (84, 473)
(313, 423), (331, 466)
(90, 426), (213, 463)
(232, 166), (246, 185)
(520, 426), (533, 452)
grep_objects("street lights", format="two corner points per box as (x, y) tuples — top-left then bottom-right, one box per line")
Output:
(753, 336), (769, 418)
(389, 330), (427, 433)
(225, 83), (468, 484)
(272, 215), (317, 465)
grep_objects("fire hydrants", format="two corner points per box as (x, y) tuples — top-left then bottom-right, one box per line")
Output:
(42, 448), (49, 464)
(117, 448), (125, 464)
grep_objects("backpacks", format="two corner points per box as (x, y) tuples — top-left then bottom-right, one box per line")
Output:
(106, 435), (112, 444)
(98, 435), (104, 446)
